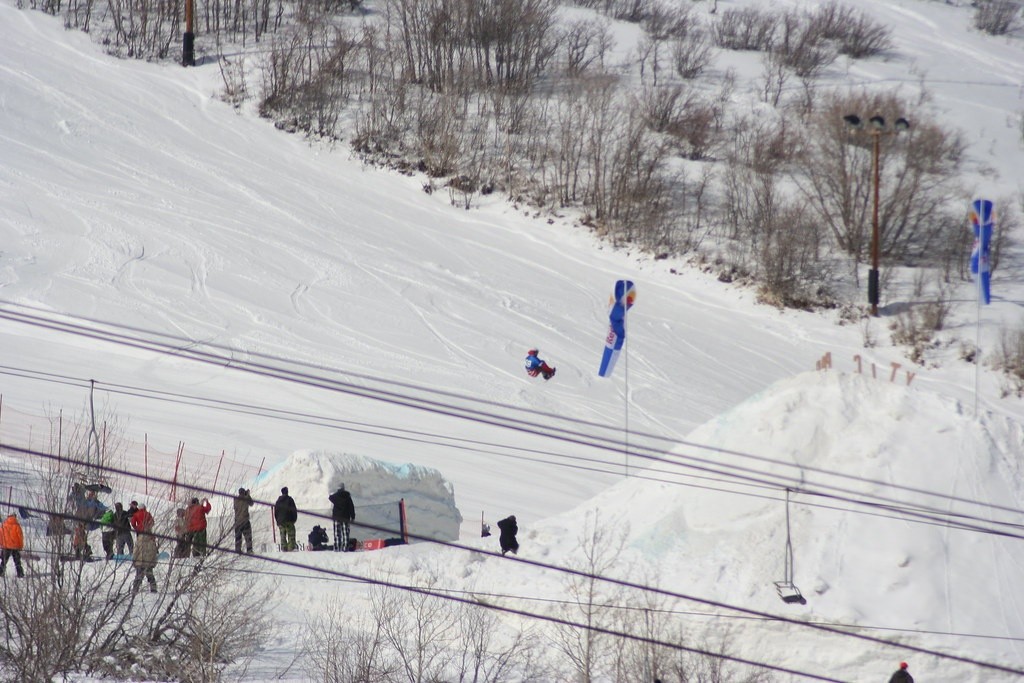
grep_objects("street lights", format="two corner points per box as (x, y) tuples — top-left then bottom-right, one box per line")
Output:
(846, 112), (909, 316)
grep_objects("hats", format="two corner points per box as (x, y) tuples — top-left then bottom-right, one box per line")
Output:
(138, 503), (145, 510)
(281, 487), (288, 494)
(338, 482), (344, 489)
(900, 662), (907, 669)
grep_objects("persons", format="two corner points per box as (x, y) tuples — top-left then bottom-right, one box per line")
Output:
(497, 515), (520, 556)
(46, 483), (155, 564)
(232, 487), (255, 554)
(188, 495), (211, 557)
(274, 486), (298, 552)
(308, 525), (335, 551)
(524, 346), (556, 381)
(130, 528), (159, 593)
(328, 481), (356, 552)
(0, 512), (25, 578)
(175, 507), (188, 558)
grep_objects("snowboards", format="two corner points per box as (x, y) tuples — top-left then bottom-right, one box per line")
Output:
(543, 366), (556, 380)
